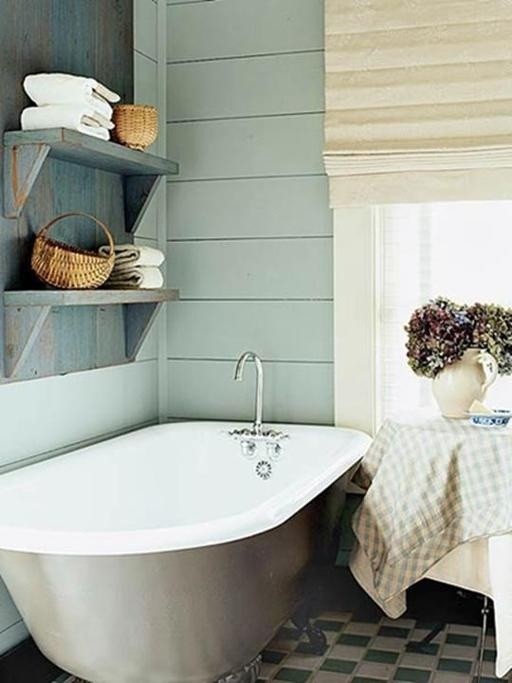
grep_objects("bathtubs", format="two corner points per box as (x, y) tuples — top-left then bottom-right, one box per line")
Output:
(0, 421), (384, 683)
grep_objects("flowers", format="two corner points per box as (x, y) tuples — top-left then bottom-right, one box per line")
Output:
(404, 295), (512, 377)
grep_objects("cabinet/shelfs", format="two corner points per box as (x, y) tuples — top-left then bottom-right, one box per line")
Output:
(2, 126), (180, 376)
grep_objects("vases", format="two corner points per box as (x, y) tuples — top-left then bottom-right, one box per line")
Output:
(431, 347), (494, 420)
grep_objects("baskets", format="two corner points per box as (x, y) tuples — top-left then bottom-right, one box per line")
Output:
(111, 103), (159, 152)
(30, 212), (117, 291)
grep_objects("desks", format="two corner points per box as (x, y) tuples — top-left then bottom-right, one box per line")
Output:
(358, 409), (509, 674)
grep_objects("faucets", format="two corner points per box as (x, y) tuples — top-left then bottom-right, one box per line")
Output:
(235, 350), (264, 432)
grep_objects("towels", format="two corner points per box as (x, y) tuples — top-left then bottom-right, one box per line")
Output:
(22, 71), (121, 141)
(100, 243), (166, 289)
